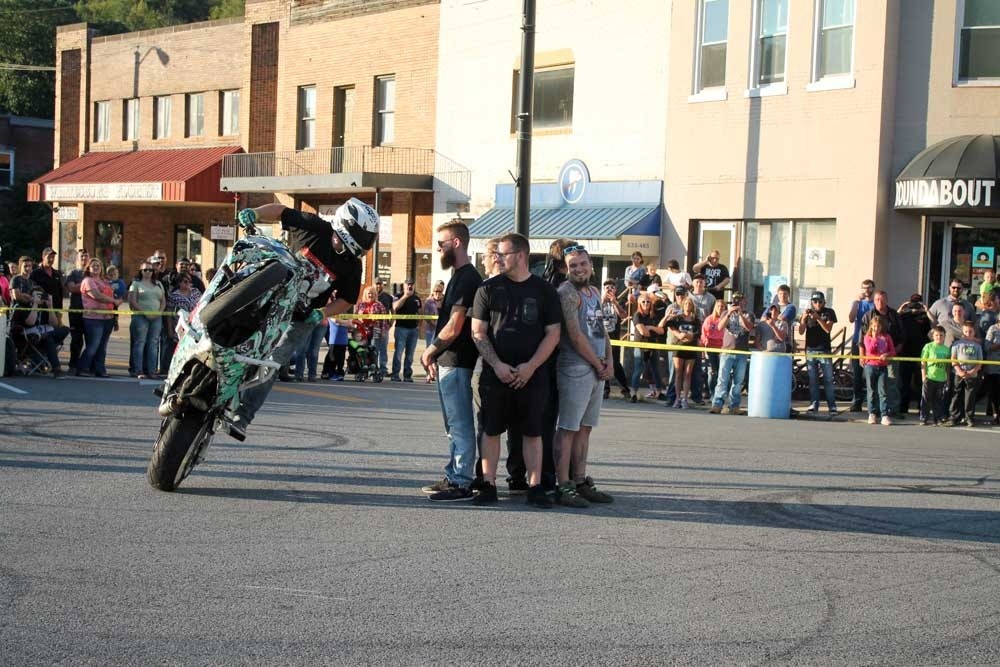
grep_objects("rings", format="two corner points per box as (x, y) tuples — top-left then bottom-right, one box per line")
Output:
(520, 380), (523, 383)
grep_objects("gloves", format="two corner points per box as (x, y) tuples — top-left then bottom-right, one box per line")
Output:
(238, 208), (258, 225)
(305, 310), (326, 326)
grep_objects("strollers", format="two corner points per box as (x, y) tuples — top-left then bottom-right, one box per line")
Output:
(345, 322), (388, 383)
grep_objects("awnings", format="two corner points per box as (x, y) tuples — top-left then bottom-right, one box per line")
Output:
(27, 145), (243, 207)
(892, 134), (1000, 217)
(466, 207), (660, 256)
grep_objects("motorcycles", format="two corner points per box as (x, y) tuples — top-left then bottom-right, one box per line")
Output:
(144, 217), (317, 495)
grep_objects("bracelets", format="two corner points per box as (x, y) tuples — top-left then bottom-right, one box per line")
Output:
(817, 318), (821, 320)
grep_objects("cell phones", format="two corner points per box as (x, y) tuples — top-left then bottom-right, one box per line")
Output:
(733, 298), (739, 306)
(672, 310), (682, 314)
(766, 313), (771, 320)
(608, 290), (612, 293)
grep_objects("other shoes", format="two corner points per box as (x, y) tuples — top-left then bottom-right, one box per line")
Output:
(231, 413), (249, 436)
(3, 360), (172, 380)
(273, 366), (436, 384)
(602, 380), (1000, 428)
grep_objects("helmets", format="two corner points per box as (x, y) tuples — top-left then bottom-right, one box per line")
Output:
(330, 197), (379, 260)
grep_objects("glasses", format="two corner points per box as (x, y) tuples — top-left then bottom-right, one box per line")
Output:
(563, 245), (585, 258)
(494, 250), (521, 261)
(437, 238), (456, 247)
(950, 286), (963, 291)
(156, 257), (166, 260)
(139, 268), (154, 273)
(676, 293), (685, 296)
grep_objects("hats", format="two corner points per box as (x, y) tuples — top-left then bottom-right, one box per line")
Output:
(602, 279), (616, 285)
(693, 273), (704, 279)
(810, 291), (824, 301)
(910, 294), (924, 303)
(374, 276), (383, 285)
(405, 278), (415, 284)
(674, 285), (686, 294)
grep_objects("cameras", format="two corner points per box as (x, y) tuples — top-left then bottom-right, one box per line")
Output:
(908, 302), (921, 309)
(808, 309), (813, 313)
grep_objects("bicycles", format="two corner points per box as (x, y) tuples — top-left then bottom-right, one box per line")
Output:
(792, 357), (856, 403)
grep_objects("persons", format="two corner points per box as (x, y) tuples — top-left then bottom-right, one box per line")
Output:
(0, 248), (217, 380)
(799, 290), (840, 416)
(274, 277), (445, 384)
(420, 220), (614, 509)
(228, 197), (380, 434)
(849, 267), (1000, 428)
(601, 249), (800, 415)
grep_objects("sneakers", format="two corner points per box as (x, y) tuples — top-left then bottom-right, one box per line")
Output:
(418, 470), (618, 510)
